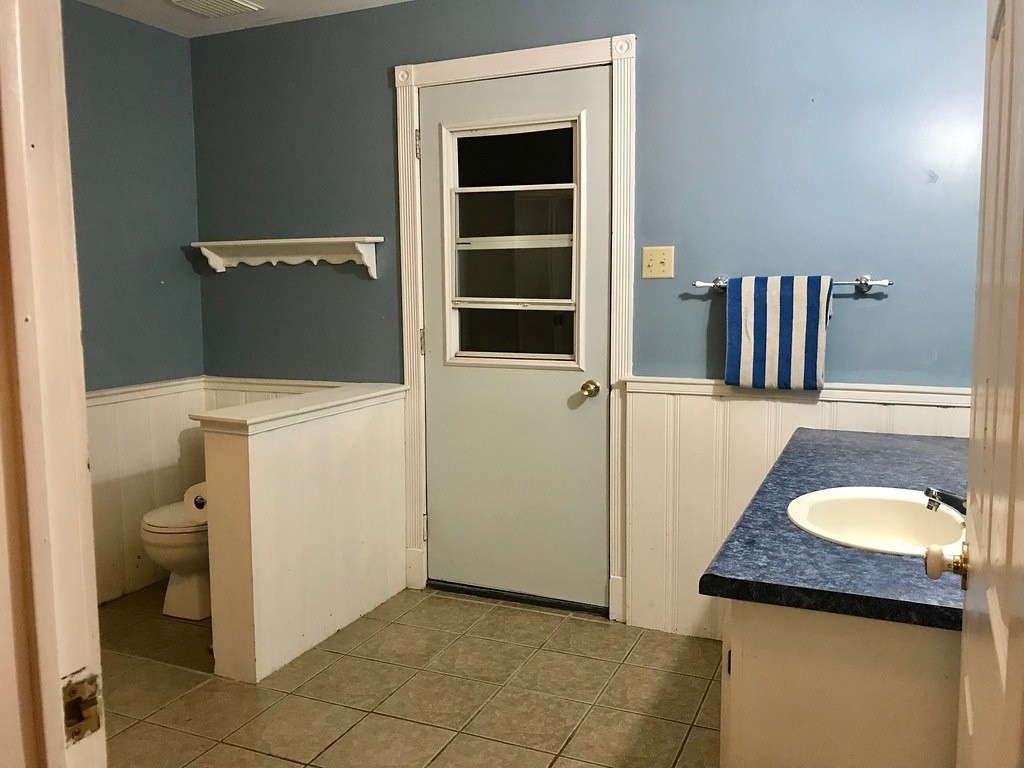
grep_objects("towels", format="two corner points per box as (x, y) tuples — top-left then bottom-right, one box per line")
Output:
(725, 275), (833, 390)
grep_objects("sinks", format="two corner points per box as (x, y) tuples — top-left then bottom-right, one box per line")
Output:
(787, 486), (968, 556)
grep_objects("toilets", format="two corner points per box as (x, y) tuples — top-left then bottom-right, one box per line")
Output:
(141, 501), (207, 619)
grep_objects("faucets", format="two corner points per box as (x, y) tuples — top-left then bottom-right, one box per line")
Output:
(925, 486), (967, 512)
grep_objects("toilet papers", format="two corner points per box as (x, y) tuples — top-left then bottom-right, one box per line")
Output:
(185, 482), (209, 524)
